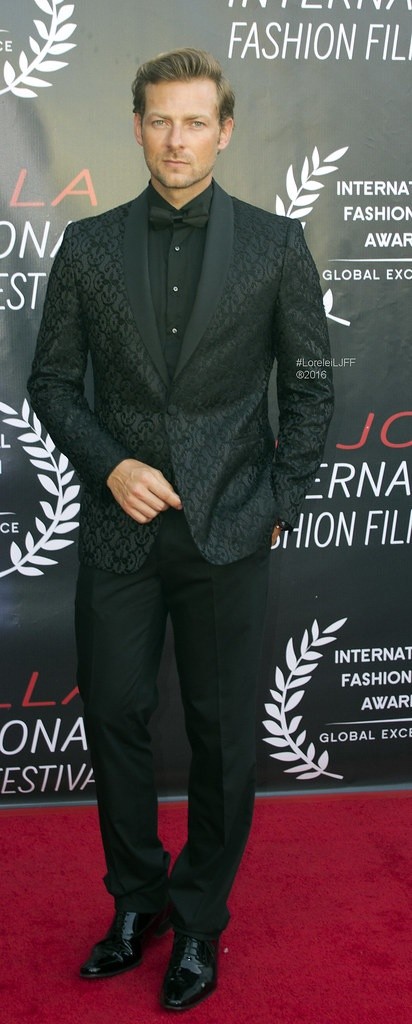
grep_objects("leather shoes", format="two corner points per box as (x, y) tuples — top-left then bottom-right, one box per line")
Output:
(159, 929), (219, 1010)
(79, 904), (175, 978)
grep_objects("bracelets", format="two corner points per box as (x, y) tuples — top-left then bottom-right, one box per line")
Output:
(277, 520), (287, 530)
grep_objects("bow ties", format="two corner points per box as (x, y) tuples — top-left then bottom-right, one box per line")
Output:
(148, 201), (209, 232)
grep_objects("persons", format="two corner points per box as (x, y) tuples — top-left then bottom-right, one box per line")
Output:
(26, 48), (334, 1010)
